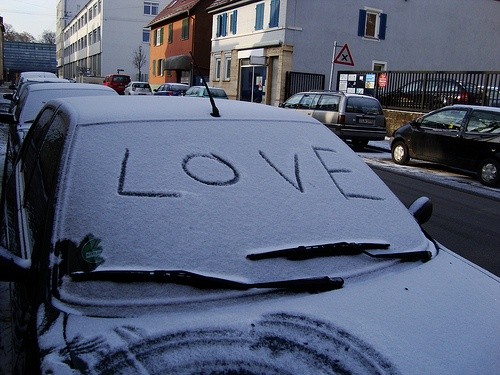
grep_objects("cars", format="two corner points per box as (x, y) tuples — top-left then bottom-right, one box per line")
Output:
(180, 86), (229, 100)
(463, 84), (500, 108)
(0, 82), (120, 166)
(0, 96), (500, 375)
(3, 71), (71, 110)
(390, 104), (500, 189)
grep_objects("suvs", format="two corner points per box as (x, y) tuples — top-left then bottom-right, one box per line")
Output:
(152, 82), (190, 96)
(124, 81), (153, 96)
(375, 78), (489, 113)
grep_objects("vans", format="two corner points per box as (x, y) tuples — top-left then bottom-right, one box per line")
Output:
(103, 74), (132, 95)
(278, 91), (387, 152)
